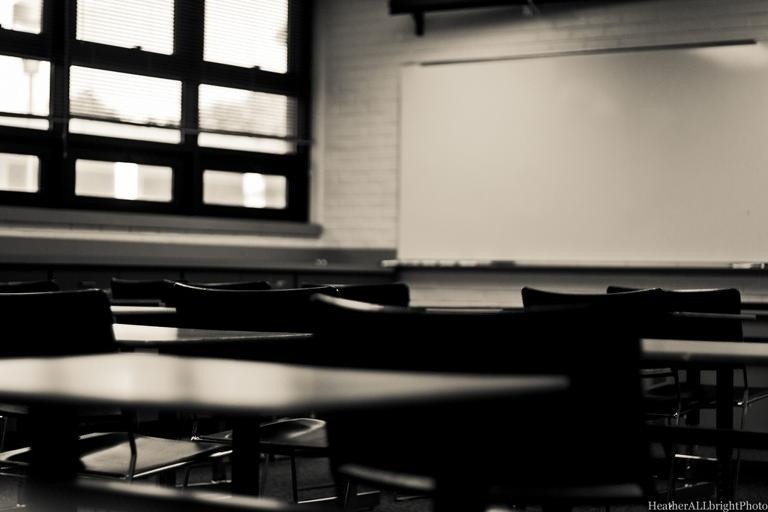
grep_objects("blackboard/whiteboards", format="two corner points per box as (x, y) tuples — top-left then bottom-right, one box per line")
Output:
(382, 39), (768, 273)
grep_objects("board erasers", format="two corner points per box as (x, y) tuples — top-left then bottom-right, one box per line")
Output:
(491, 260), (514, 266)
